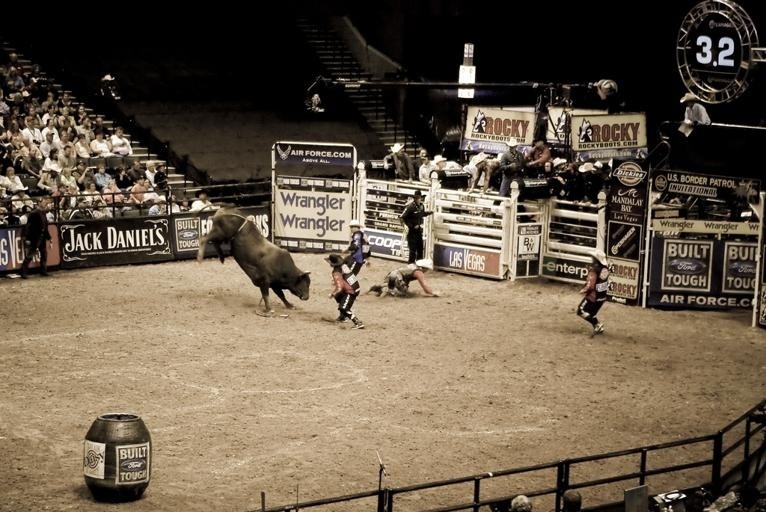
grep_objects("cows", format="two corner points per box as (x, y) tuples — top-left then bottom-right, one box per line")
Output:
(197, 203), (311, 313)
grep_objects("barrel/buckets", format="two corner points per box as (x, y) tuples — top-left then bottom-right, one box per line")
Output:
(82, 412), (151, 504)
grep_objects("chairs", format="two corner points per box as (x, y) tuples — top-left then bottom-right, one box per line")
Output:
(14, 155), (149, 217)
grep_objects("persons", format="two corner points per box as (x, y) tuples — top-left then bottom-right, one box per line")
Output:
(324, 254), (364, 330)
(400, 190), (435, 265)
(366, 258), (440, 297)
(511, 489), (583, 512)
(680, 92), (711, 125)
(342, 220), (371, 276)
(383, 139), (611, 247)
(0, 52), (209, 279)
(576, 251), (611, 333)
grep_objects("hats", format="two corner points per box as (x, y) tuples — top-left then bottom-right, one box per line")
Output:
(390, 143), (405, 156)
(410, 190), (427, 198)
(587, 249), (608, 267)
(414, 258), (434, 270)
(344, 220), (364, 229)
(552, 157), (567, 166)
(579, 163), (597, 173)
(323, 255), (343, 266)
(506, 136), (519, 147)
(431, 154), (448, 164)
(468, 153), (488, 168)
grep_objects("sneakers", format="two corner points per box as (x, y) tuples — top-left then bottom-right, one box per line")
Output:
(353, 323), (365, 329)
(337, 317), (348, 323)
(592, 324), (605, 337)
